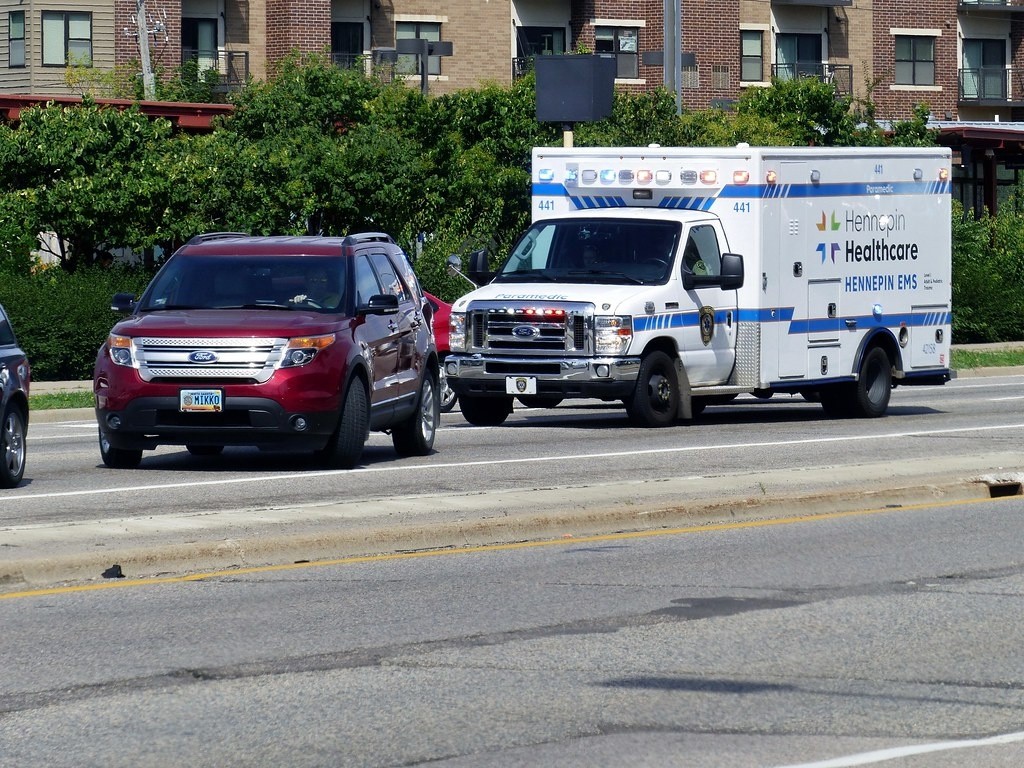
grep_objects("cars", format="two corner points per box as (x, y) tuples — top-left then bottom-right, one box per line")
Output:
(421, 287), (462, 410)
(0, 305), (30, 484)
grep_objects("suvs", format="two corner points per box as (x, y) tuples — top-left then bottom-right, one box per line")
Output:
(93, 226), (443, 468)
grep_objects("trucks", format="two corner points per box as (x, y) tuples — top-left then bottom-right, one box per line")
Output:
(445, 131), (954, 427)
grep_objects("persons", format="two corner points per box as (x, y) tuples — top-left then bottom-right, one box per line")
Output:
(293, 265), (338, 307)
(683, 237), (713, 275)
(582, 247), (598, 266)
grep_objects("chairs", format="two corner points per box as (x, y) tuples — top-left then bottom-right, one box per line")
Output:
(202, 269), (248, 308)
